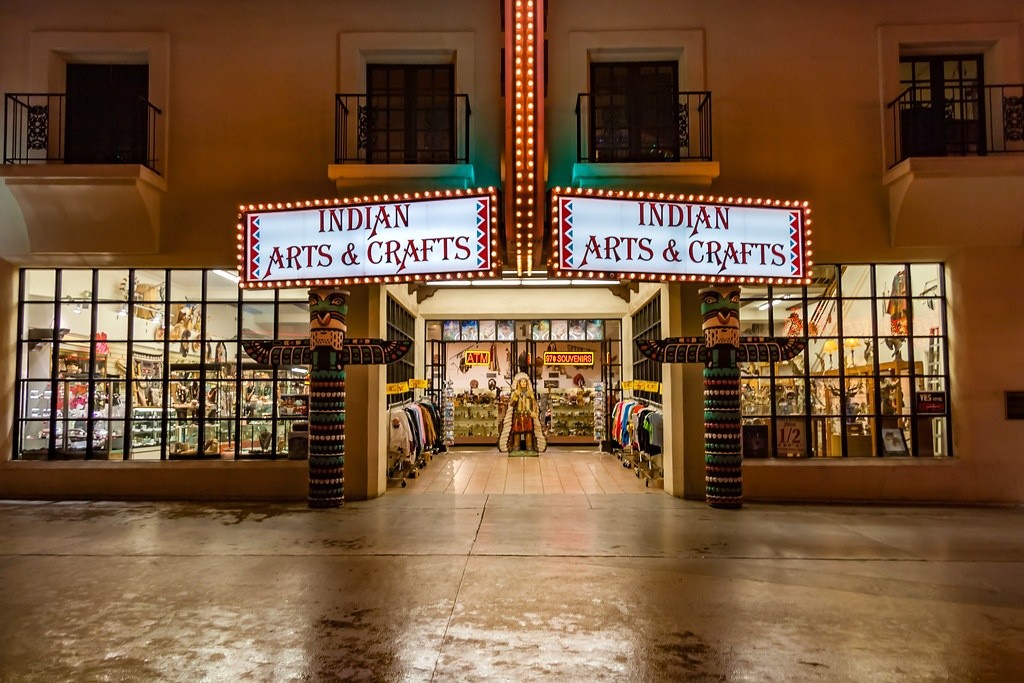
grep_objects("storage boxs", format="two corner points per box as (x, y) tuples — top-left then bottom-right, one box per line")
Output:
(916, 389), (944, 413)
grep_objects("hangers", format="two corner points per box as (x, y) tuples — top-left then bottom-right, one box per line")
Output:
(898, 263), (906, 276)
(388, 394), (433, 410)
(622, 394), (664, 415)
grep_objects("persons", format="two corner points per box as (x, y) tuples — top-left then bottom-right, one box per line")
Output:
(496, 372), (547, 456)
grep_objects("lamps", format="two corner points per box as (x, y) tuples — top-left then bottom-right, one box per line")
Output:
(844, 338), (862, 368)
(822, 339), (838, 371)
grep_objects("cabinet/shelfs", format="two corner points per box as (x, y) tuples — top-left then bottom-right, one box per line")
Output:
(452, 387), (600, 444)
(741, 359), (925, 457)
(22, 344), (309, 456)
